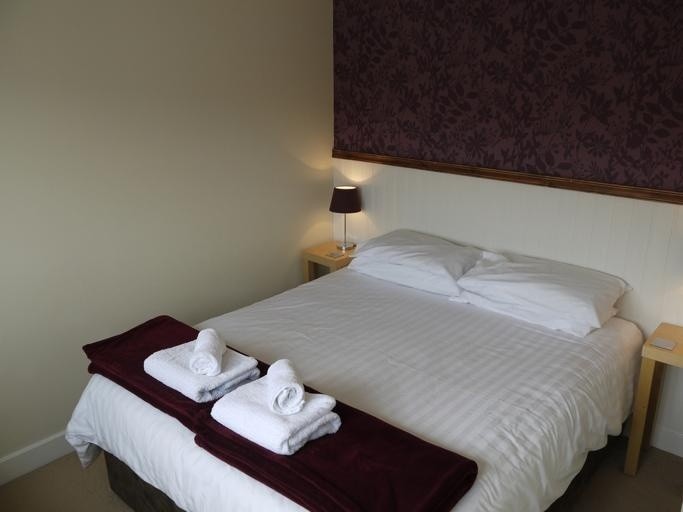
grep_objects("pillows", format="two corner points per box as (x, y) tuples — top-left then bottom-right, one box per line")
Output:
(349, 229), (634, 338)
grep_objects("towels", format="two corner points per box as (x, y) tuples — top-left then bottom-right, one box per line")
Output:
(143, 328), (341, 456)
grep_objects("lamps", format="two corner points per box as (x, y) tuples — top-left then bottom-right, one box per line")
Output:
(329, 186), (361, 250)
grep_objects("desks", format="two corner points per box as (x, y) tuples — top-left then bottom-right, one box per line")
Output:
(623, 321), (683, 477)
(301, 241), (356, 282)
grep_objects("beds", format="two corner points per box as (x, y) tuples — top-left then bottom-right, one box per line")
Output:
(94, 229), (642, 512)
